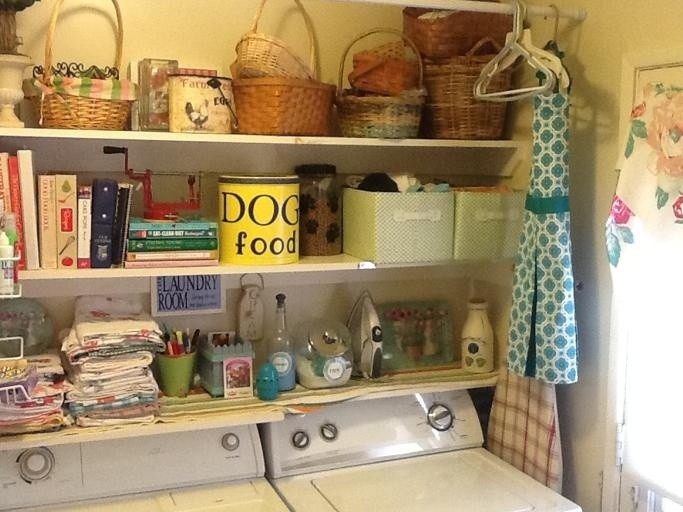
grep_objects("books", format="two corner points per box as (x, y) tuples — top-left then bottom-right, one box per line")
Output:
(0, 147), (221, 273)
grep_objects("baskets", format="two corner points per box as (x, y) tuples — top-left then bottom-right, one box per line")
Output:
(21, 0), (138, 131)
(232, 0), (317, 80)
(402, 0), (531, 59)
(229, 76), (339, 137)
(421, 36), (513, 141)
(334, 26), (429, 139)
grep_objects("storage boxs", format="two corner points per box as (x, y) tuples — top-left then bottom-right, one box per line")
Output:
(343, 167), (528, 266)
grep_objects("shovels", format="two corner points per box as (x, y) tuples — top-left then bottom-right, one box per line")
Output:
(206, 79), (238, 122)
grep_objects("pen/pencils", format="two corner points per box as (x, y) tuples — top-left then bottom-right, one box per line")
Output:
(164, 324), (201, 356)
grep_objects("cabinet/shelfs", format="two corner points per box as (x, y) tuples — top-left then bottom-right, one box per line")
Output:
(0, 127), (531, 447)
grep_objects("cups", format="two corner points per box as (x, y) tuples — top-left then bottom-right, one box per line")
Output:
(155, 344), (197, 398)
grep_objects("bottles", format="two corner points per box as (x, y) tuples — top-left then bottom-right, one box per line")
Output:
(255, 293), (354, 401)
(460, 297), (493, 374)
(0, 231), (15, 296)
(294, 163), (342, 257)
